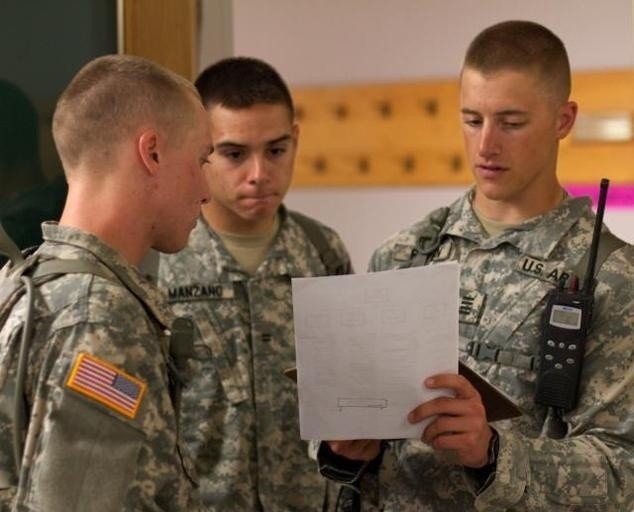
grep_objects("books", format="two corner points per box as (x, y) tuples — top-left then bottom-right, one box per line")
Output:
(282, 359), (524, 423)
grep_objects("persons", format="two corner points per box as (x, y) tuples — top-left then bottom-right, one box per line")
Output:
(1, 54), (214, 511)
(307, 19), (634, 511)
(137, 56), (356, 512)
